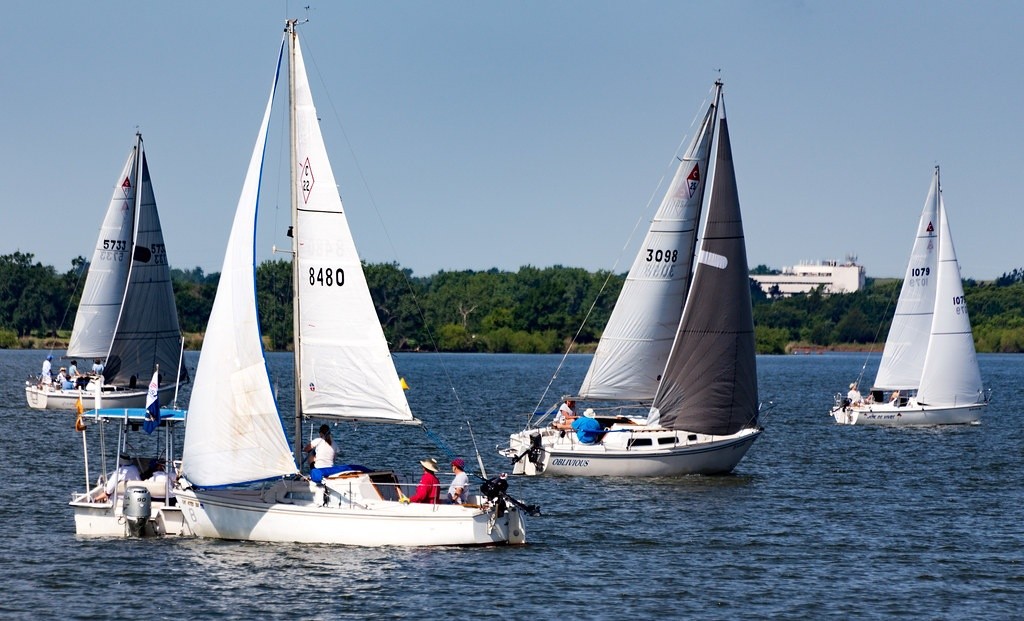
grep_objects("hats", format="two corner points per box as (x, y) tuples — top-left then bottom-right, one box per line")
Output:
(849, 383), (856, 389)
(119, 453), (133, 466)
(60, 366), (66, 371)
(419, 458), (440, 472)
(451, 459), (465, 467)
(583, 408), (596, 418)
(47, 355), (53, 360)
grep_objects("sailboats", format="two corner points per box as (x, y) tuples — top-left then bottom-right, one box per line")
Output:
(23, 124), (193, 412)
(494, 65), (769, 482)
(828, 165), (990, 426)
(174, 16), (541, 548)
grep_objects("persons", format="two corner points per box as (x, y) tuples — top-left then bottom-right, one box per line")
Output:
(68, 360), (82, 376)
(571, 408), (600, 445)
(847, 383), (865, 406)
(554, 400), (575, 429)
(42, 355), (53, 388)
(866, 391), (884, 402)
(55, 368), (67, 381)
(91, 358), (104, 374)
(406, 459), (440, 504)
(93, 452), (141, 501)
(60, 375), (75, 390)
(446, 458), (469, 504)
(890, 390), (899, 402)
(305, 425), (340, 470)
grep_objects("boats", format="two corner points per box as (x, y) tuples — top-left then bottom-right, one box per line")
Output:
(67, 407), (195, 538)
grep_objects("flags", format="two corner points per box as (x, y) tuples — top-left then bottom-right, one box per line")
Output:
(401, 377), (409, 390)
(142, 369), (161, 434)
